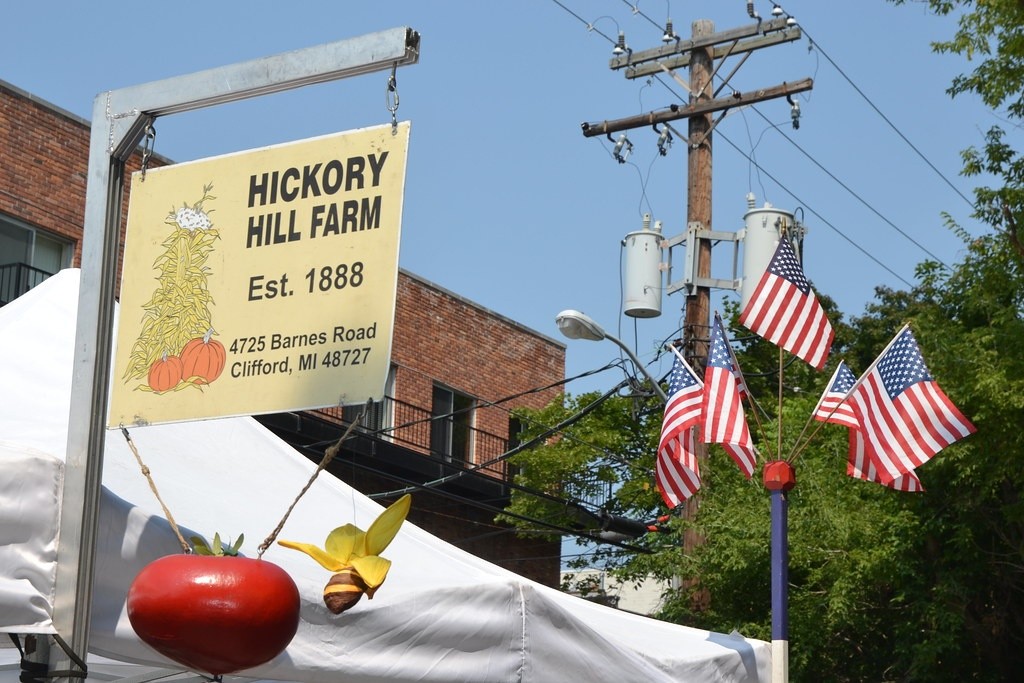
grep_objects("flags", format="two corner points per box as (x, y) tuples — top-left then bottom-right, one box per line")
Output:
(738, 231), (835, 373)
(812, 359), (927, 491)
(699, 314), (756, 481)
(657, 352), (704, 510)
(845, 324), (978, 486)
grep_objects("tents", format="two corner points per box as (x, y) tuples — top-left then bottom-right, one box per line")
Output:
(0, 268), (772, 683)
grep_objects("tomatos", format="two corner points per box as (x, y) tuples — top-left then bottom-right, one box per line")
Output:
(126, 533), (300, 677)
